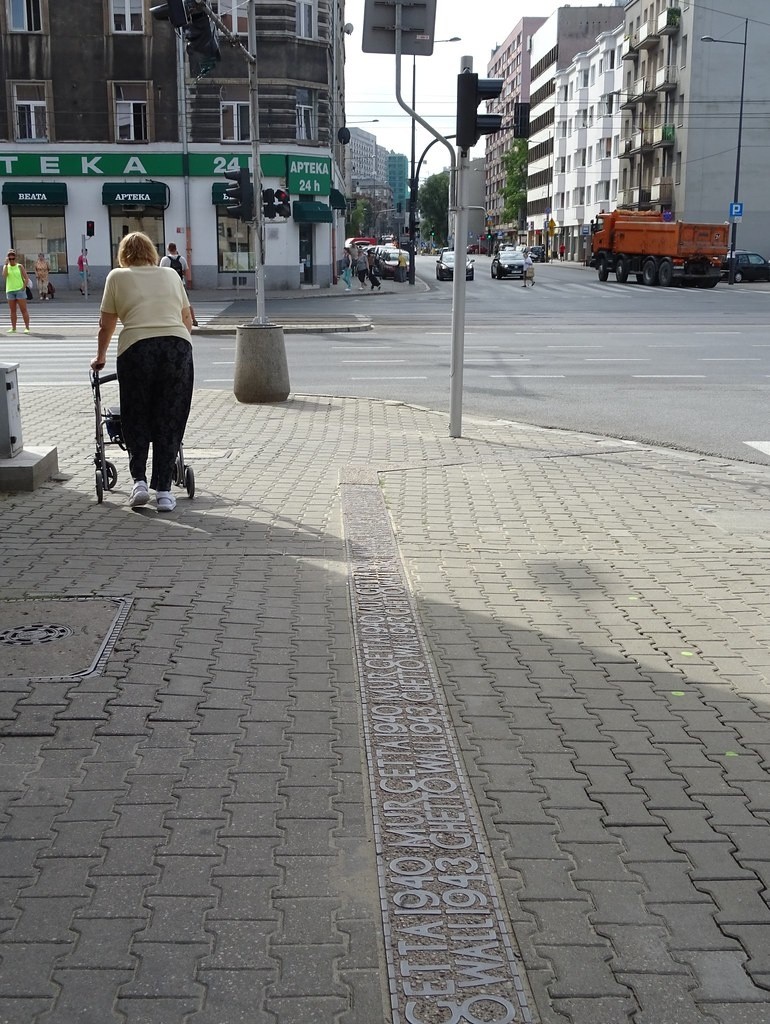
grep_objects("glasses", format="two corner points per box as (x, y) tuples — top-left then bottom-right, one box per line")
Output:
(9, 257), (16, 261)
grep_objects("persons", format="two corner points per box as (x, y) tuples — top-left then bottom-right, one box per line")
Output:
(366, 250), (374, 280)
(397, 251), (407, 283)
(34, 252), (50, 300)
(356, 245), (362, 252)
(559, 242), (566, 263)
(77, 248), (91, 295)
(160, 243), (198, 326)
(367, 252), (381, 290)
(342, 247), (352, 291)
(495, 241), (499, 256)
(2, 249), (30, 333)
(356, 249), (370, 290)
(431, 239), (436, 256)
(392, 239), (398, 248)
(349, 243), (358, 278)
(421, 240), (428, 256)
(521, 251), (535, 287)
(370, 235), (376, 245)
(378, 237), (385, 245)
(90, 231), (194, 511)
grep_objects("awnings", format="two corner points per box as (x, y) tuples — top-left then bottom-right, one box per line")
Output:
(2, 181), (68, 205)
(102, 182), (167, 206)
(329, 189), (346, 210)
(293, 201), (333, 223)
(212, 182), (263, 205)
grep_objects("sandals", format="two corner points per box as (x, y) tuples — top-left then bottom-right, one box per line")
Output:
(129, 481), (150, 507)
(156, 491), (177, 511)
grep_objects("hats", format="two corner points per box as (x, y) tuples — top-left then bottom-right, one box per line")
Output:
(7, 249), (16, 256)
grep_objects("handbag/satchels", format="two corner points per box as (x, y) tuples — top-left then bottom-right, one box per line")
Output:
(23, 279), (33, 300)
(47, 282), (55, 294)
(526, 263), (535, 278)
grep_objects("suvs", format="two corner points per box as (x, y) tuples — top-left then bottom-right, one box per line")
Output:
(720, 248), (770, 283)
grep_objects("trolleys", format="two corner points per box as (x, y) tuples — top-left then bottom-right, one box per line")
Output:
(90, 362), (196, 502)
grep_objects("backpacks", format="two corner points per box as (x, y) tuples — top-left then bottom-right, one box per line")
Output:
(167, 255), (183, 280)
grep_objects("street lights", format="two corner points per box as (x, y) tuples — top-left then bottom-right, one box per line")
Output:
(700, 17), (749, 286)
(526, 130), (550, 263)
(474, 168), (492, 257)
(410, 36), (461, 178)
(608, 78), (645, 211)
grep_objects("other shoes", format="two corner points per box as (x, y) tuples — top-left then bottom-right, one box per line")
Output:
(46, 297), (49, 300)
(522, 285), (526, 287)
(80, 288), (82, 293)
(6, 328), (17, 333)
(24, 328), (31, 333)
(377, 284), (381, 290)
(344, 288), (351, 291)
(82, 292), (90, 296)
(358, 286), (365, 290)
(193, 321), (198, 326)
(371, 285), (374, 290)
(39, 297), (44, 300)
(531, 281), (536, 286)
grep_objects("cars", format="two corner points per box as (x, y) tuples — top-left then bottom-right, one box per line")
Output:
(345, 237), (410, 280)
(467, 244), (487, 254)
(498, 243), (543, 263)
(490, 251), (527, 280)
(435, 252), (475, 281)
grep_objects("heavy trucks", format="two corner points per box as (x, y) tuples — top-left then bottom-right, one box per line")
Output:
(589, 208), (730, 289)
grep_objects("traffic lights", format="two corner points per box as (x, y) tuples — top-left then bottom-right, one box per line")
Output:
(456, 72), (505, 147)
(414, 221), (420, 233)
(182, 12), (221, 66)
(86, 221), (94, 236)
(487, 229), (491, 239)
(148, 0), (187, 29)
(275, 188), (291, 216)
(224, 167), (253, 222)
(430, 231), (435, 235)
